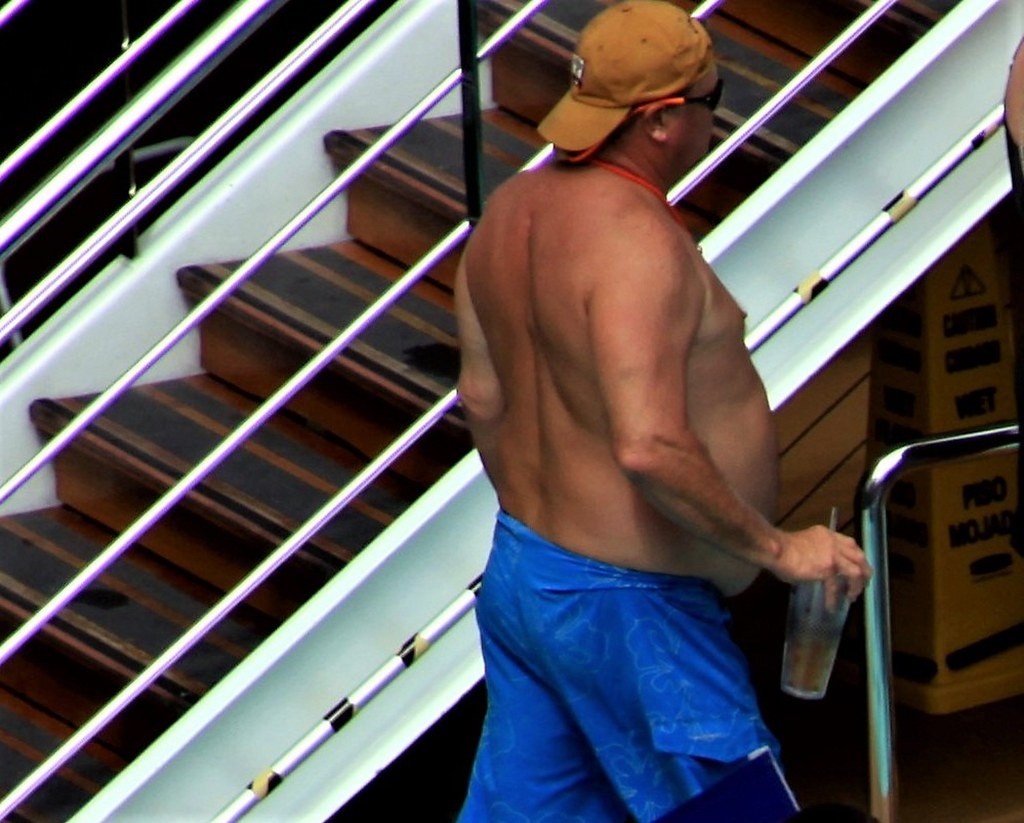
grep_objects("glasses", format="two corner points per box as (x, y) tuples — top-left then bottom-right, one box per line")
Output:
(630, 77), (724, 115)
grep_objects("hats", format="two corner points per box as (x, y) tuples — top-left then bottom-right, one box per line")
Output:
(536, 1), (718, 149)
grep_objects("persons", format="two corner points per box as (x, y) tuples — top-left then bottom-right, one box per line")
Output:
(454, 0), (871, 822)
(1002, 32), (1024, 574)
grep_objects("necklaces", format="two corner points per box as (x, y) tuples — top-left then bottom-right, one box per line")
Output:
(589, 158), (687, 232)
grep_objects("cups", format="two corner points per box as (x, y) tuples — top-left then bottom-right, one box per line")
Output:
(779, 580), (854, 699)
